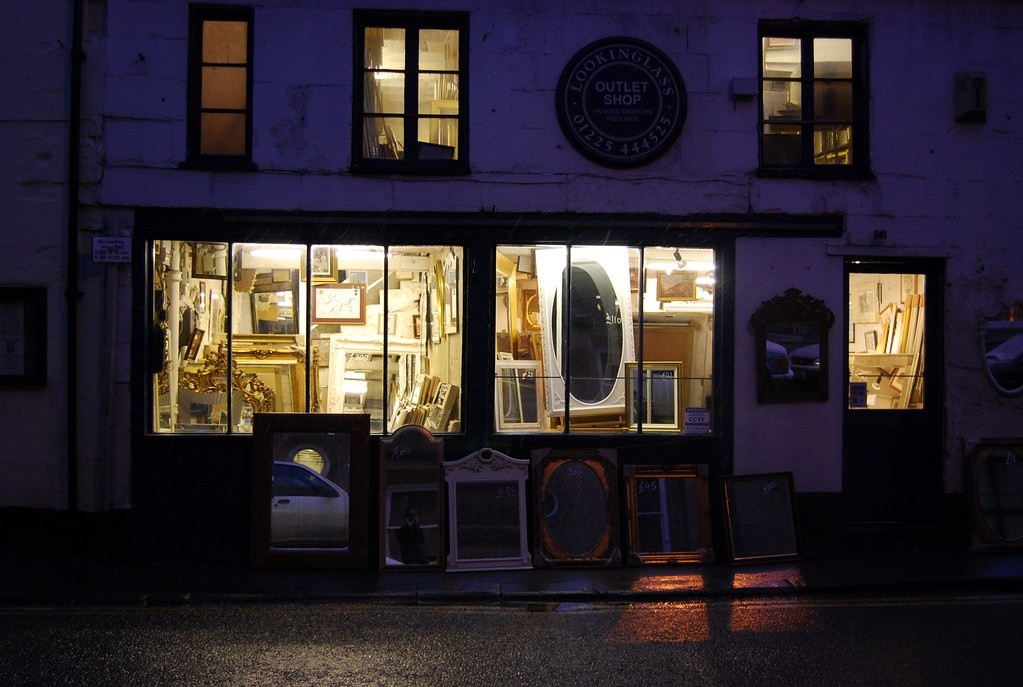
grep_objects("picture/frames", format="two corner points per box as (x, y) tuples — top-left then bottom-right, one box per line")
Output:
(749, 287), (834, 406)
(715, 470), (808, 567)
(623, 463), (719, 567)
(182, 240), (698, 434)
(530, 447), (621, 569)
(765, 37), (801, 51)
(848, 271), (925, 409)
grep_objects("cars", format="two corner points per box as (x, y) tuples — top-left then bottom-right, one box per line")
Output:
(270, 458), (349, 544)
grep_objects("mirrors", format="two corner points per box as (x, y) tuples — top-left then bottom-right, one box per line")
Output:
(532, 246), (636, 415)
(249, 412), (372, 575)
(443, 447), (535, 573)
(379, 425), (447, 573)
(328, 332), (423, 436)
(496, 250), (519, 363)
(249, 280), (301, 336)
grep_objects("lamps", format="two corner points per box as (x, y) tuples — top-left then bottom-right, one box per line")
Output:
(670, 247), (687, 268)
